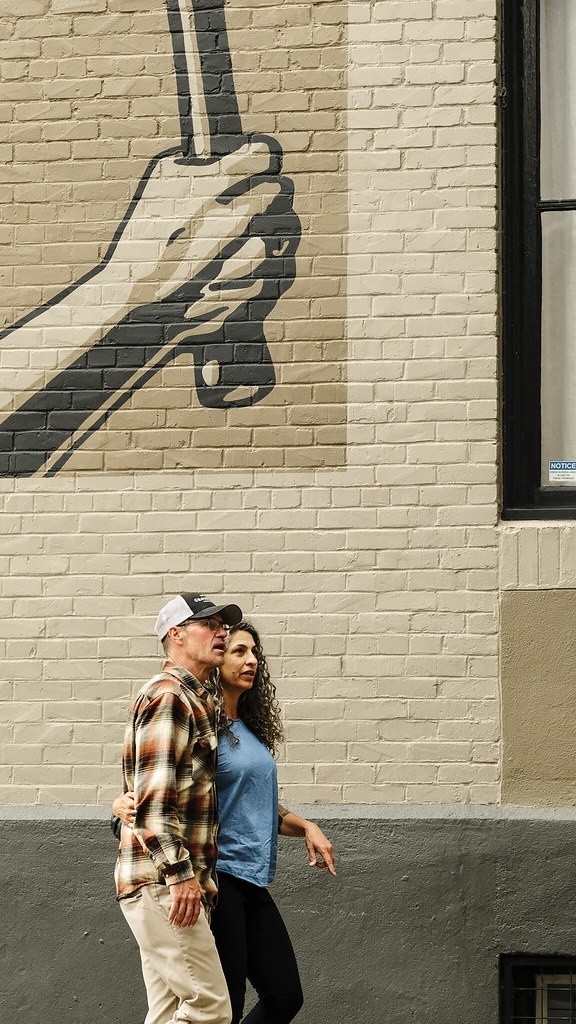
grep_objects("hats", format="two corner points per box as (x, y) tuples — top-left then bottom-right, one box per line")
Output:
(154, 592), (243, 642)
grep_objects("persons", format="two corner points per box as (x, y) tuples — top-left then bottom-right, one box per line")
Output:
(112, 616), (337, 1023)
(111, 592), (245, 1024)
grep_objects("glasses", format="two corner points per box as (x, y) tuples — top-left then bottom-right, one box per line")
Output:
(175, 618), (230, 632)
